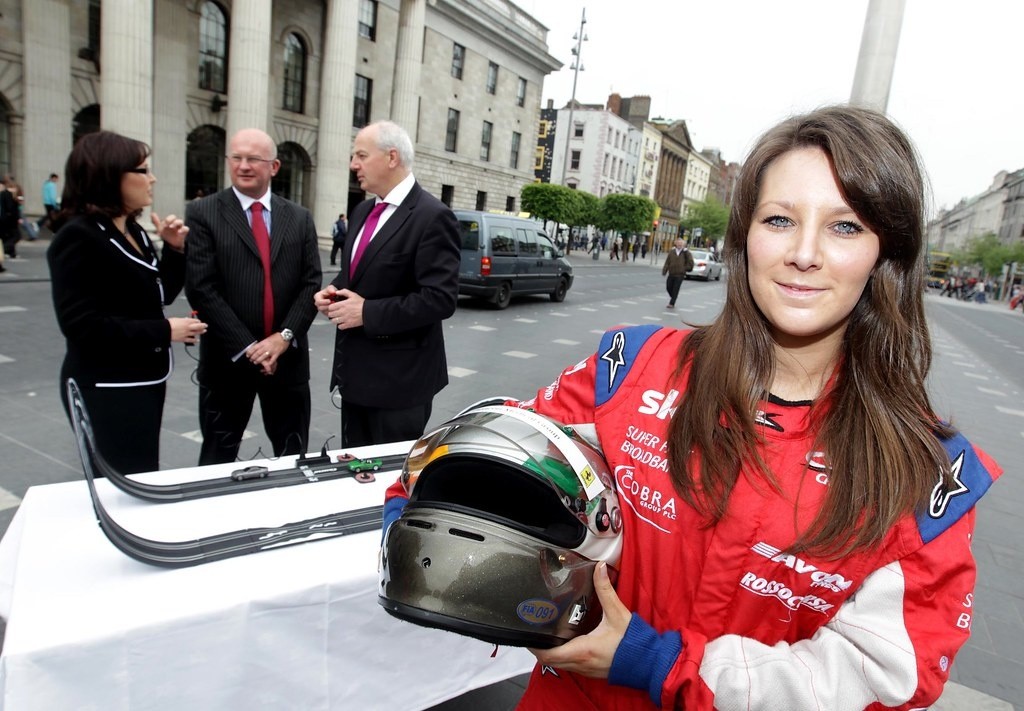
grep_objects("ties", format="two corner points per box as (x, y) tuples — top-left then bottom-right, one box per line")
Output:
(251, 202), (275, 338)
(349, 203), (387, 281)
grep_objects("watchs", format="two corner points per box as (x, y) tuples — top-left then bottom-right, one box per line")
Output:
(278, 327), (294, 343)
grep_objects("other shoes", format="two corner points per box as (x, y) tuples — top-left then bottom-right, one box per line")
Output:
(667, 304), (674, 308)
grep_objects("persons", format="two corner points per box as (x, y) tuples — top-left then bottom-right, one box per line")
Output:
(47, 129), (208, 480)
(315, 119), (462, 453)
(941, 274), (1024, 310)
(661, 239), (694, 309)
(377, 109), (1003, 711)
(0, 174), (64, 273)
(194, 189), (202, 201)
(557, 229), (659, 261)
(185, 129), (321, 467)
(330, 214), (348, 266)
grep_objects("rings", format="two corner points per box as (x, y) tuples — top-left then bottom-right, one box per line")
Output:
(265, 352), (269, 356)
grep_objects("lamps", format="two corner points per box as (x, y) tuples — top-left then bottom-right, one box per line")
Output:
(209, 95), (227, 113)
(79, 47), (100, 75)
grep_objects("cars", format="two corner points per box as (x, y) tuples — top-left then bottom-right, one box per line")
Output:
(686, 251), (723, 281)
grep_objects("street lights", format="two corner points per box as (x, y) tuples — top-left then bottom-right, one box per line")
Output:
(560, 8), (588, 187)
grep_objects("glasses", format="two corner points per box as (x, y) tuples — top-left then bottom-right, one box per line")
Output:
(230, 154), (274, 164)
(127, 166), (152, 175)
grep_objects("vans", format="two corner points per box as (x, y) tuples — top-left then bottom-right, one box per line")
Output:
(451, 209), (574, 310)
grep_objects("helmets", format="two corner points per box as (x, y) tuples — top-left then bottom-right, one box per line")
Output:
(379, 406), (623, 650)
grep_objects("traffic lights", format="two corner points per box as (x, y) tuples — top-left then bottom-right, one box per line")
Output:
(653, 221), (658, 231)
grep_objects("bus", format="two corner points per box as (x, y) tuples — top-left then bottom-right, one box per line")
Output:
(925, 251), (957, 289)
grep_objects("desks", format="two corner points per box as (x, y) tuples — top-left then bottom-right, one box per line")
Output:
(0, 439), (538, 711)
